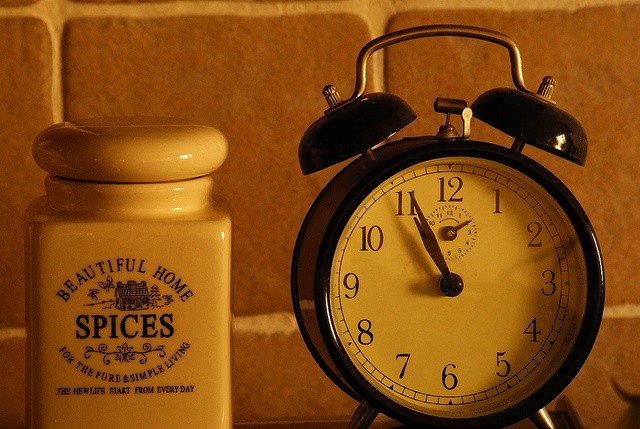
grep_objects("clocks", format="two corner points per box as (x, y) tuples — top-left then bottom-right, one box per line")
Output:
(289, 22), (607, 426)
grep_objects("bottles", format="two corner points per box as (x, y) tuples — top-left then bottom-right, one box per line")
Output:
(23, 117), (232, 429)
